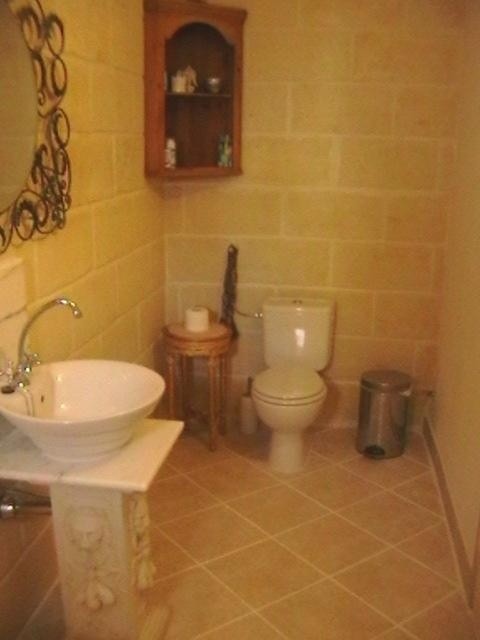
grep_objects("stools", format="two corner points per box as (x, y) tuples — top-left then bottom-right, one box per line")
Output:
(162, 319), (234, 449)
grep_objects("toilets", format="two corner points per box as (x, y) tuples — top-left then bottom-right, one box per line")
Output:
(251, 297), (335, 473)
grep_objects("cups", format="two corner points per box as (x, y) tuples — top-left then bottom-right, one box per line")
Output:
(170, 76), (188, 93)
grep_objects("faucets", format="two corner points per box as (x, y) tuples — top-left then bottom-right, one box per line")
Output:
(18, 298), (82, 366)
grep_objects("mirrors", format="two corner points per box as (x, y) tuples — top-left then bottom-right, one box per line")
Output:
(0, 0), (84, 252)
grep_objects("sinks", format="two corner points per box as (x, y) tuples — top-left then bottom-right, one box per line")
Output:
(0, 358), (166, 460)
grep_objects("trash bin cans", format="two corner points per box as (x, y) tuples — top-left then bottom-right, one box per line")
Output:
(356, 369), (414, 460)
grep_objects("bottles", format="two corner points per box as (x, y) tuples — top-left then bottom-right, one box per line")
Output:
(165, 138), (177, 169)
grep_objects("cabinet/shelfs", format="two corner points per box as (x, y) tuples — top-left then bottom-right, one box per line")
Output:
(143, 0), (245, 185)
